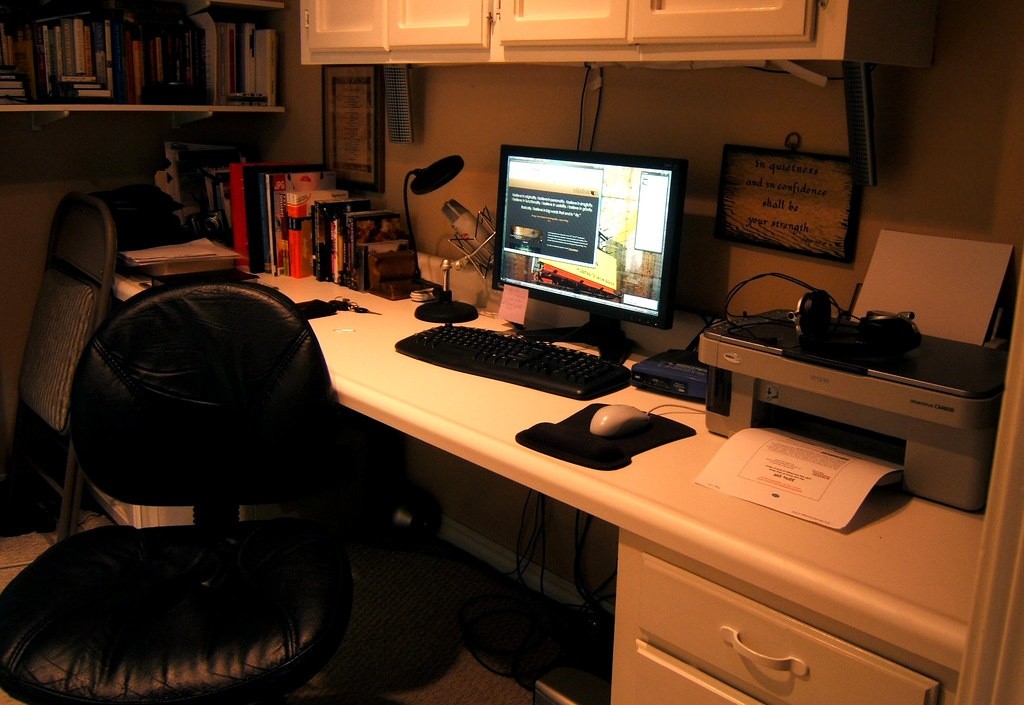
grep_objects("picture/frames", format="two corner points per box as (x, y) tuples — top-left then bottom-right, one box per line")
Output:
(320, 65), (387, 195)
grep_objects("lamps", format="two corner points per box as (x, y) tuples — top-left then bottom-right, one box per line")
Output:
(402, 158), (479, 322)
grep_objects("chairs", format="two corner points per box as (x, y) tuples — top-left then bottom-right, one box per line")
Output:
(0, 191), (120, 594)
(0, 279), (354, 705)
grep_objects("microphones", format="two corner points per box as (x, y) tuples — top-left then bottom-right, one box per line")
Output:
(441, 200), (495, 266)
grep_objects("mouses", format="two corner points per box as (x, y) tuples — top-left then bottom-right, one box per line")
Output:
(589, 404), (652, 435)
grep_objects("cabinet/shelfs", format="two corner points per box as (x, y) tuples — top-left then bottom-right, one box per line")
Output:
(0, 0), (287, 113)
(111, 268), (987, 705)
(298, 0), (938, 70)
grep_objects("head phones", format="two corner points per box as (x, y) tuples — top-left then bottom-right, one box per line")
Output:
(723, 273), (922, 356)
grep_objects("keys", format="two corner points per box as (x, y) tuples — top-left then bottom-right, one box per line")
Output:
(331, 300), (381, 314)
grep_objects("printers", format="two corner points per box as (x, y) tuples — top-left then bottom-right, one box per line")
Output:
(696, 255), (1018, 513)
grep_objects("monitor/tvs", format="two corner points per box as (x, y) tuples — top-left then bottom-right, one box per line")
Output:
(491, 145), (688, 367)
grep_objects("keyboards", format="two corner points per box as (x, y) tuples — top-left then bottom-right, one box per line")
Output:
(394, 325), (632, 401)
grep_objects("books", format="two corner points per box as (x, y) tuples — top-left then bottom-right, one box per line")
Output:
(230, 161), (403, 291)
(0, 9), (278, 105)
